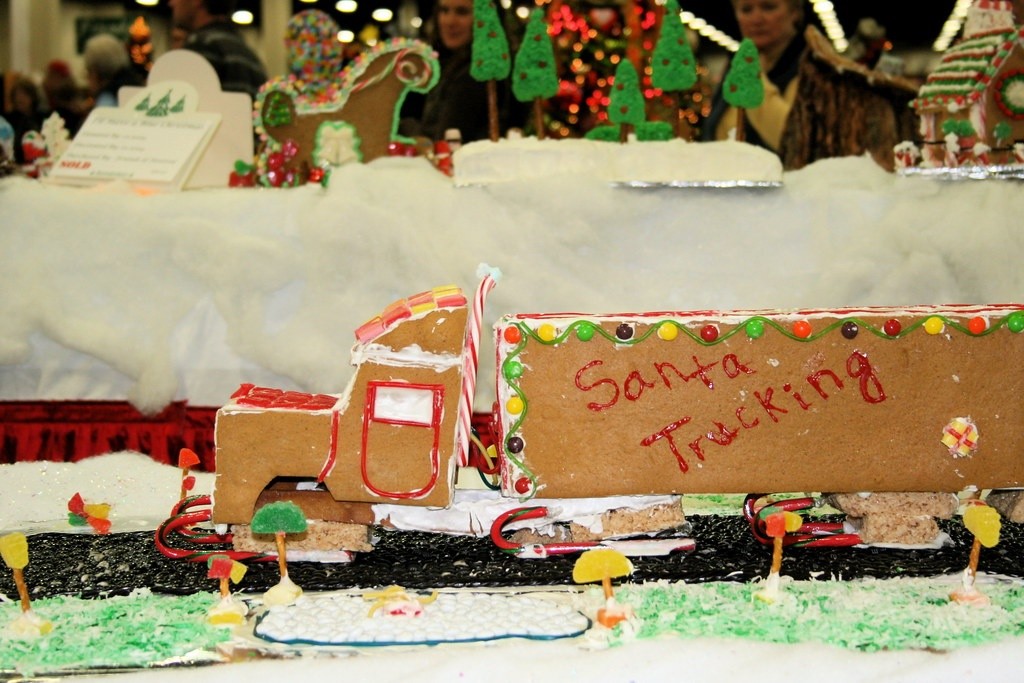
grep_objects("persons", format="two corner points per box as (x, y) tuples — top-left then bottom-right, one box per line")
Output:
(403, 0), (533, 152)
(701, 0), (814, 154)
(0, 0), (267, 179)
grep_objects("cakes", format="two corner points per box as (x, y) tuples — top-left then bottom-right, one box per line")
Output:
(1, 0), (1024, 603)
